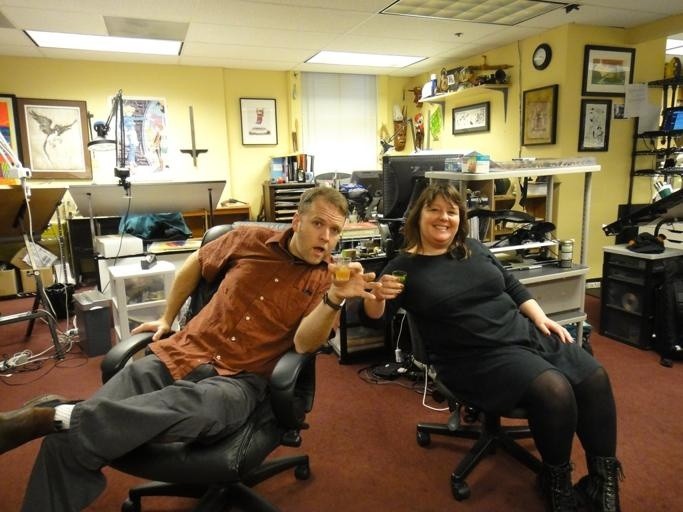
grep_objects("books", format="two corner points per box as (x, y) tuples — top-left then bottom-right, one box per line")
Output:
(269, 153), (315, 182)
(341, 222), (381, 235)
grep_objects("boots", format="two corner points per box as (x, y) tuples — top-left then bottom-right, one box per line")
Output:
(535, 467), (576, 512)
(573, 458), (626, 512)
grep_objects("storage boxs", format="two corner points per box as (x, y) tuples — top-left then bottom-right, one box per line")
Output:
(12, 244), (59, 270)
(0, 268), (21, 296)
(21, 265), (54, 293)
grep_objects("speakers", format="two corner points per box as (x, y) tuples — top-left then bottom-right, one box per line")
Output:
(598, 243), (683, 350)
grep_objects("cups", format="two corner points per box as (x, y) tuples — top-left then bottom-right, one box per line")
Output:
(392, 270), (408, 283)
(334, 257), (350, 280)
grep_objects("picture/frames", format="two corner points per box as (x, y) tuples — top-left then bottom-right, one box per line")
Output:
(14, 97), (93, 182)
(579, 99), (612, 151)
(521, 84), (557, 147)
(0, 94), (24, 186)
(583, 46), (637, 99)
(239, 98), (279, 144)
(452, 101), (490, 135)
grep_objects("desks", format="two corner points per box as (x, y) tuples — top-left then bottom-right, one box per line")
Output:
(427, 165), (601, 353)
(314, 251), (396, 364)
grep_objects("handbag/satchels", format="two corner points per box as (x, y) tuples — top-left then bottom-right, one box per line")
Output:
(120, 210), (192, 242)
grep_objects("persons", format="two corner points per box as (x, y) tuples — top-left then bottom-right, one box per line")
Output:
(0, 187), (383, 511)
(360, 179), (622, 512)
(249, 107), (272, 136)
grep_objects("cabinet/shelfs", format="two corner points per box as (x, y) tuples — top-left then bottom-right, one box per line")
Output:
(263, 181), (317, 223)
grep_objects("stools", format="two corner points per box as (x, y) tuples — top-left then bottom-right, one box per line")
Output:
(109, 261), (177, 345)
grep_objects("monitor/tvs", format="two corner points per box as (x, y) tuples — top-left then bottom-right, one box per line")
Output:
(384, 156), (467, 219)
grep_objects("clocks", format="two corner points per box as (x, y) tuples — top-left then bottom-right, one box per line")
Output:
(532, 43), (551, 70)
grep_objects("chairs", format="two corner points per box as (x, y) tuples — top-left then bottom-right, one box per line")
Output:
(396, 305), (544, 502)
(101, 226), (319, 512)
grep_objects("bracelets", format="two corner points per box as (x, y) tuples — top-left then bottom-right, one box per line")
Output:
(320, 288), (348, 312)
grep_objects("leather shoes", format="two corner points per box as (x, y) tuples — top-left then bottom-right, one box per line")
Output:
(0, 393), (63, 457)
